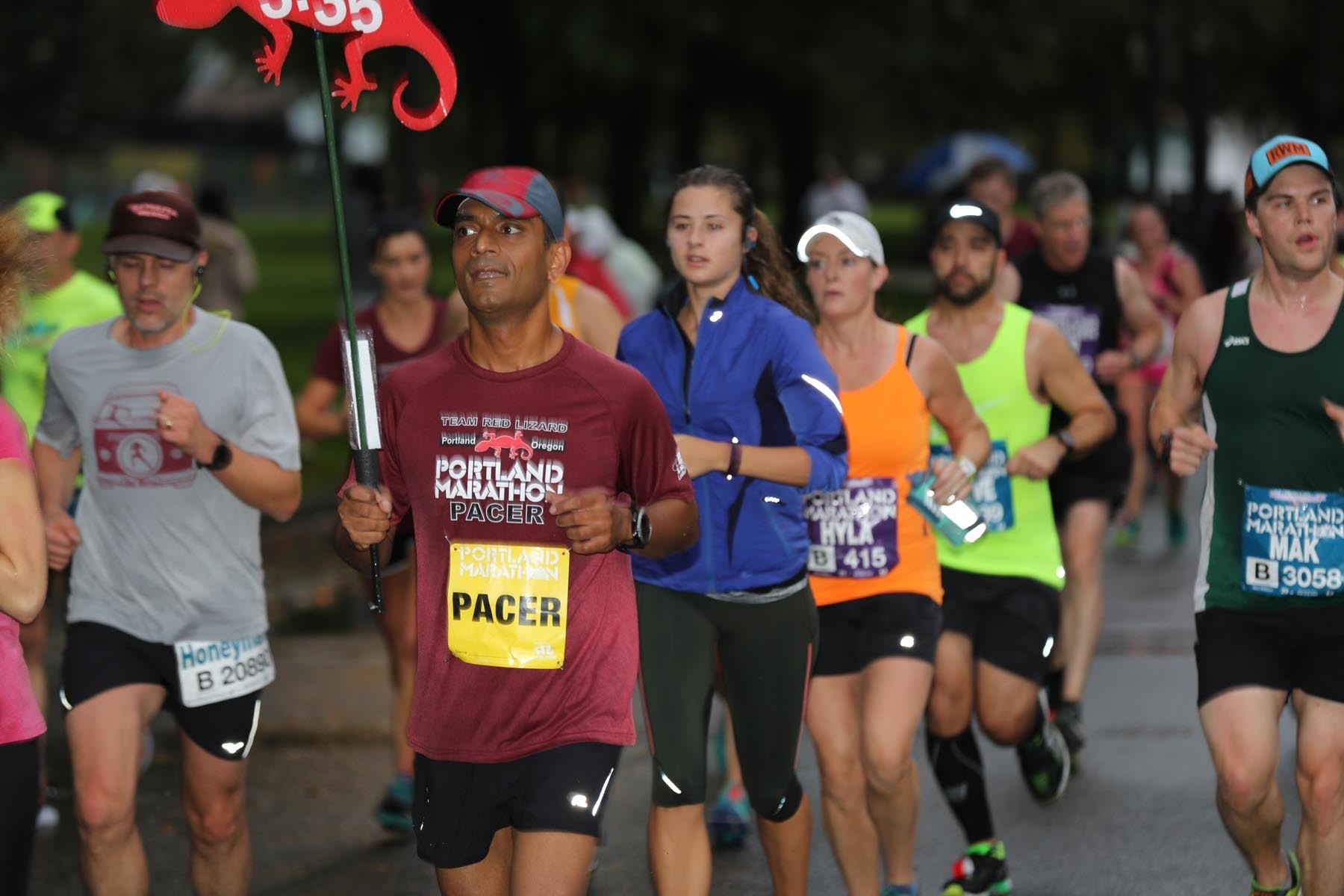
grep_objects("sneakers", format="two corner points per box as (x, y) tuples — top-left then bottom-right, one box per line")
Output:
(1053, 696), (1084, 777)
(940, 839), (1013, 896)
(1017, 688), (1071, 805)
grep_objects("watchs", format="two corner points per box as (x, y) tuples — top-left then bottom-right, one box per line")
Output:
(615, 499), (652, 551)
(196, 432), (232, 471)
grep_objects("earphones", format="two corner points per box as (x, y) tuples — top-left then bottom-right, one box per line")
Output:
(197, 265), (205, 280)
(106, 267), (117, 281)
(745, 236), (750, 250)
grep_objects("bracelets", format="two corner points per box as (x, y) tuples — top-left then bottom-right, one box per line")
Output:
(724, 437), (743, 479)
(1156, 431), (1173, 472)
(951, 455), (977, 483)
(1056, 430), (1075, 456)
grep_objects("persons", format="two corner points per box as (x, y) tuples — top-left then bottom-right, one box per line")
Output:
(0, 135), (1344, 896)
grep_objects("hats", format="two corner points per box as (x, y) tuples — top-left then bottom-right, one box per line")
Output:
(103, 188), (202, 263)
(16, 191), (74, 233)
(1242, 133), (1333, 206)
(434, 167), (563, 246)
(935, 197), (1002, 248)
(796, 210), (885, 267)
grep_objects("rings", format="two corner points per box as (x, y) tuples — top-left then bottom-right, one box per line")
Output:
(166, 418), (172, 431)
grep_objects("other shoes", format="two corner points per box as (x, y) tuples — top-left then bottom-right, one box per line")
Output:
(882, 881), (920, 896)
(709, 781), (757, 850)
(374, 777), (416, 834)
(1249, 849), (1303, 896)
(36, 803), (60, 828)
